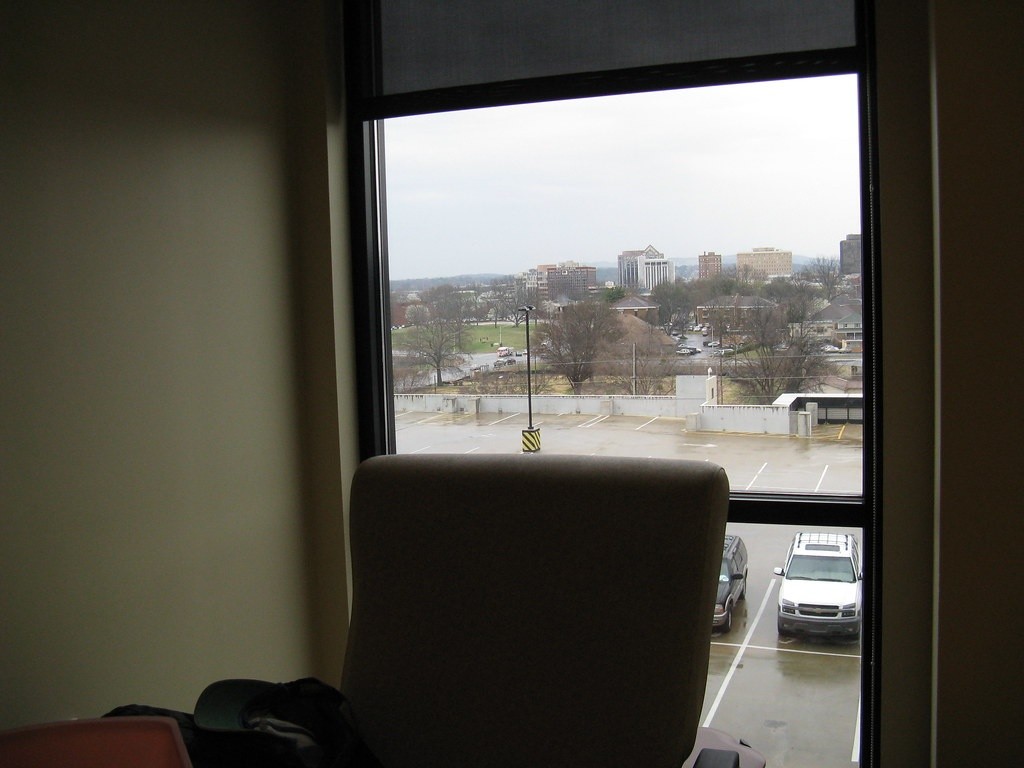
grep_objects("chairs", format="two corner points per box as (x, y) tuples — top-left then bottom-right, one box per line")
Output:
(340, 454), (742, 768)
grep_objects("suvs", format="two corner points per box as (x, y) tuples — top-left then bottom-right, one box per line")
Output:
(710, 533), (749, 637)
(773, 532), (862, 642)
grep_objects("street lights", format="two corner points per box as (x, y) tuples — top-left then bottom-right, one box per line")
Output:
(518, 305), (542, 453)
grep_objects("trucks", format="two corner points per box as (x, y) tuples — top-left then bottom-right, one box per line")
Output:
(497, 347), (514, 357)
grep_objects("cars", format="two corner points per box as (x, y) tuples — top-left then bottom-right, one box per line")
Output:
(821, 344), (852, 354)
(493, 359), (516, 367)
(516, 351), (522, 356)
(658, 323), (734, 357)
(522, 349), (527, 355)
